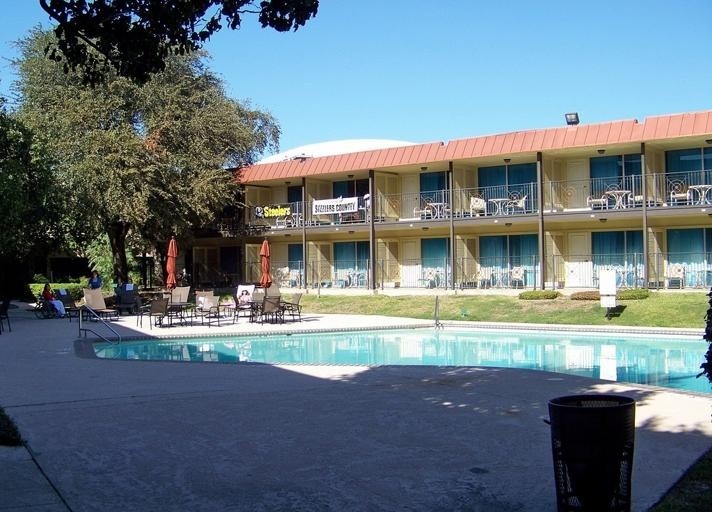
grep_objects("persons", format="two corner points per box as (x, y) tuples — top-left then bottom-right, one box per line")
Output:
(41, 282), (68, 319)
(221, 298), (235, 311)
(86, 269), (104, 289)
(239, 289), (253, 306)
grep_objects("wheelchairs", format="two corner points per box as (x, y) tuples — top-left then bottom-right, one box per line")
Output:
(34, 293), (69, 319)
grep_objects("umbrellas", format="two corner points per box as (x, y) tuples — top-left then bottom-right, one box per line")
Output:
(165, 233), (180, 306)
(257, 235), (272, 298)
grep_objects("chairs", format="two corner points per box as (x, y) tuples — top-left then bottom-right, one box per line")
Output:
(54, 283), (303, 331)
(333, 269), (350, 289)
(417, 267), (437, 289)
(280, 269), (300, 288)
(671, 183), (694, 206)
(587, 183), (643, 211)
(473, 266), (525, 290)
(469, 194), (527, 218)
(633, 264), (644, 288)
(414, 197), (434, 220)
(0, 295), (12, 335)
(665, 262), (684, 290)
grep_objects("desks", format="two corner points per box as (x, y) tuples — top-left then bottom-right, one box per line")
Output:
(426, 203), (448, 220)
(688, 185), (712, 204)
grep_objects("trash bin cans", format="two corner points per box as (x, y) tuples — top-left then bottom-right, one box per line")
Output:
(547, 395), (634, 512)
